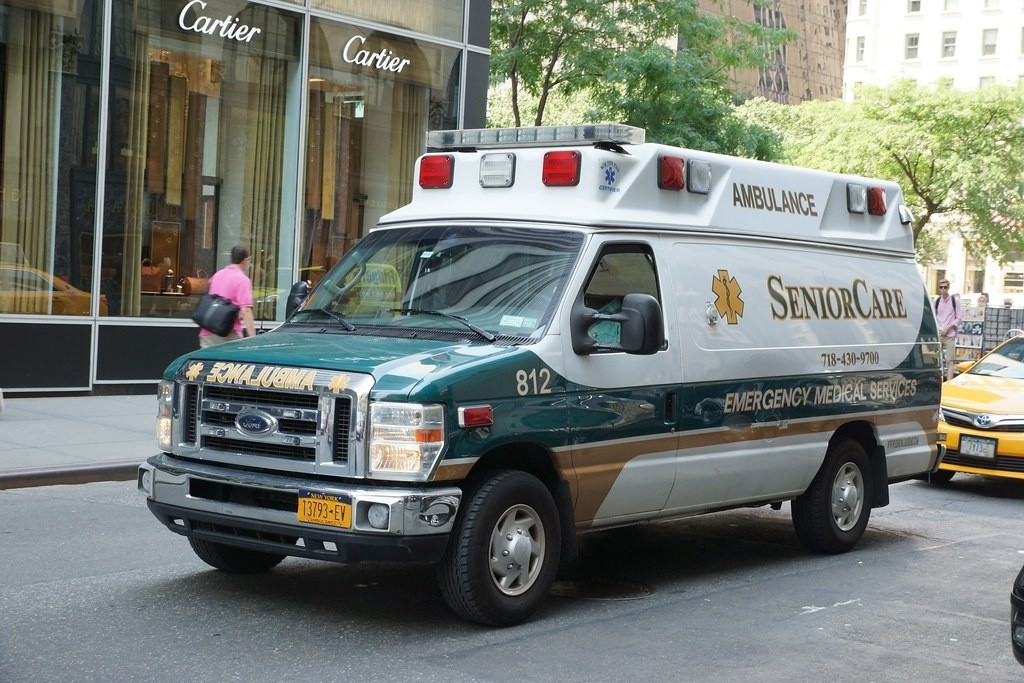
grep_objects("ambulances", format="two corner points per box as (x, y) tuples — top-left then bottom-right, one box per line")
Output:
(134, 123), (946, 628)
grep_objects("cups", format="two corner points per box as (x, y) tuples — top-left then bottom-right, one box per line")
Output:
(166, 275), (175, 291)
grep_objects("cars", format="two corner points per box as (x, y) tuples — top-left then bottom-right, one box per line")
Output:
(914, 333), (1024, 489)
(0, 263), (109, 318)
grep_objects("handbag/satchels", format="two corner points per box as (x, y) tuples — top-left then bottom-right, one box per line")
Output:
(192, 294), (241, 337)
(184, 269), (210, 297)
(141, 258), (161, 292)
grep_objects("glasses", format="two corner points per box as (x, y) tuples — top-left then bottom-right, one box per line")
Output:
(243, 257), (251, 261)
(939, 286), (948, 289)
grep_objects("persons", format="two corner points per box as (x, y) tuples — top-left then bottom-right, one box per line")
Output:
(977, 292), (1013, 309)
(931, 278), (963, 381)
(198, 245), (256, 348)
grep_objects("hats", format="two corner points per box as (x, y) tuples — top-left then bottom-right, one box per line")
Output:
(1004, 298), (1012, 304)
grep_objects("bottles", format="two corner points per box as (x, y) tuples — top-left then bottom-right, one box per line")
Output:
(177, 285), (180, 292)
(178, 286), (182, 292)
